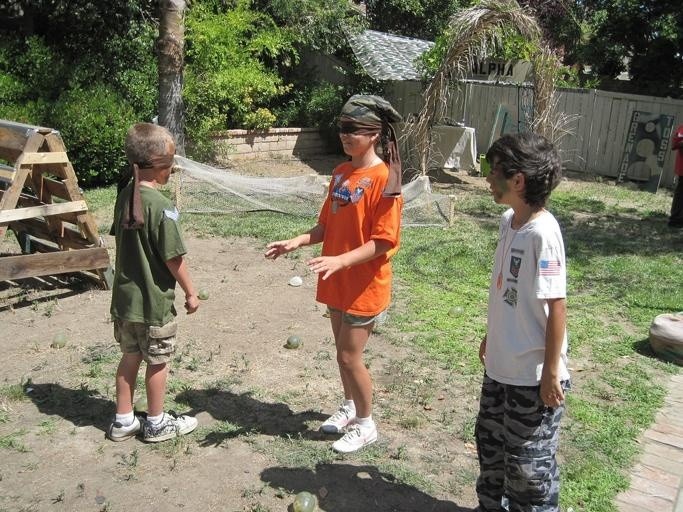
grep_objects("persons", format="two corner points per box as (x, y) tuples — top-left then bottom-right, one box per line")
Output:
(668, 123), (683, 227)
(107, 122), (198, 443)
(474, 130), (571, 511)
(263, 93), (406, 452)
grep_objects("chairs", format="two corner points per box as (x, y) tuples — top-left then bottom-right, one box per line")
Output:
(409, 113), (457, 126)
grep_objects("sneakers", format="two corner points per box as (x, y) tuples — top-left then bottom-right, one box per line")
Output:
(332, 422), (379, 453)
(321, 405), (356, 435)
(108, 416), (145, 442)
(143, 413), (197, 442)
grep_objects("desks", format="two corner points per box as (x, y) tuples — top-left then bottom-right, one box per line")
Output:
(393, 121), (478, 169)
